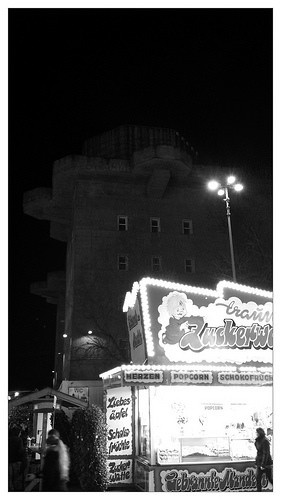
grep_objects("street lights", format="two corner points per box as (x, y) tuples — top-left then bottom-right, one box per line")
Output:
(208, 173), (244, 284)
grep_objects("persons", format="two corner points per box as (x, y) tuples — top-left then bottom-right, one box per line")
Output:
(41, 430), (69, 491)
(254, 428), (273, 492)
(9, 427), (28, 491)
